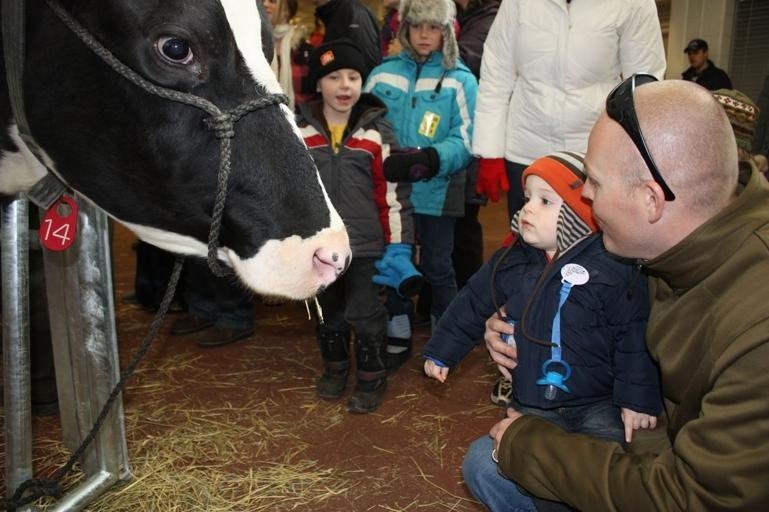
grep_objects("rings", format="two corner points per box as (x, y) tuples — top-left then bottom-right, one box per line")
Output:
(491, 449), (498, 462)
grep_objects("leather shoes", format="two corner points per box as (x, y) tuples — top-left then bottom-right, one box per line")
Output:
(149, 297), (187, 313)
(170, 315), (214, 334)
(196, 324), (256, 346)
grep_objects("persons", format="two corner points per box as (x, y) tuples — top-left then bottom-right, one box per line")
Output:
(381, 2), (460, 55)
(472, 0), (667, 224)
(291, 15), (325, 115)
(414, 1), (501, 327)
(295, 38), (415, 412)
(463, 79), (767, 511)
(171, 2), (275, 345)
(264, 2), (305, 112)
(309, 2), (382, 73)
(423, 150), (660, 511)
(682, 39), (733, 91)
(711, 89), (767, 177)
(361, 1), (478, 374)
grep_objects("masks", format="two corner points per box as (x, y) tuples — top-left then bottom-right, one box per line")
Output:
(308, 31), (323, 49)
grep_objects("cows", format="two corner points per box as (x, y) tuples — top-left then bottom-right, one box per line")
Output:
(0, 0), (352, 302)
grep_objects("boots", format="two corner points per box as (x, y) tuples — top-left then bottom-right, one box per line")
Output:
(315, 329), (352, 401)
(387, 313), (414, 373)
(345, 328), (388, 413)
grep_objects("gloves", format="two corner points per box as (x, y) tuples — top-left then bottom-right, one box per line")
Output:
(475, 158), (510, 204)
(372, 255), (424, 300)
(381, 244), (414, 271)
(383, 147), (442, 184)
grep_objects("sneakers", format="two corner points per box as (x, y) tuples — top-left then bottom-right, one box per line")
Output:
(490, 371), (515, 406)
(120, 292), (142, 310)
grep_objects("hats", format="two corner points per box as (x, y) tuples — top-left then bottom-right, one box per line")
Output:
(308, 36), (367, 93)
(709, 88), (761, 152)
(397, 3), (461, 71)
(684, 39), (708, 53)
(489, 148), (600, 348)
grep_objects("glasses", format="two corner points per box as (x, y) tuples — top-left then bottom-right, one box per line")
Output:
(606, 73), (676, 202)
